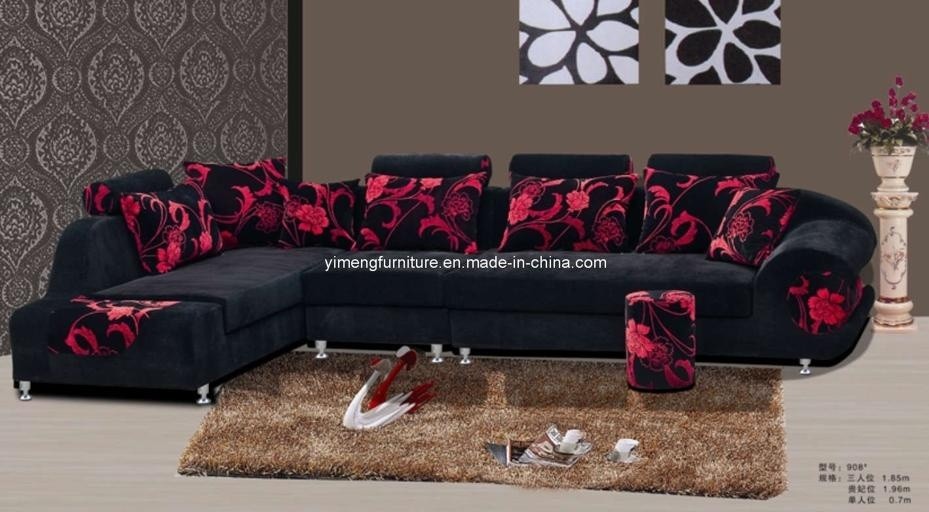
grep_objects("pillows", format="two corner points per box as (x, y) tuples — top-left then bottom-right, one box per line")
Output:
(117, 154), (800, 275)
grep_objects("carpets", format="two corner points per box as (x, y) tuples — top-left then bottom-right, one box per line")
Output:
(178, 351), (788, 500)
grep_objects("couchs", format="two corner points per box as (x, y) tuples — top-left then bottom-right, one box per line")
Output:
(9, 154), (877, 405)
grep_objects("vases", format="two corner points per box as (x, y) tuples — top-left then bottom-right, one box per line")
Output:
(870, 146), (917, 192)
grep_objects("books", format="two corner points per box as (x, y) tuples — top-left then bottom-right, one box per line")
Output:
(505, 437), (537, 470)
(518, 423), (594, 467)
(485, 441), (509, 465)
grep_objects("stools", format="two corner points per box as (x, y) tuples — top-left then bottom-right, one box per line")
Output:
(626, 290), (696, 392)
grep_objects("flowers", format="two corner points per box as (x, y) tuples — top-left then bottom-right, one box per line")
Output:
(847, 76), (929, 155)
